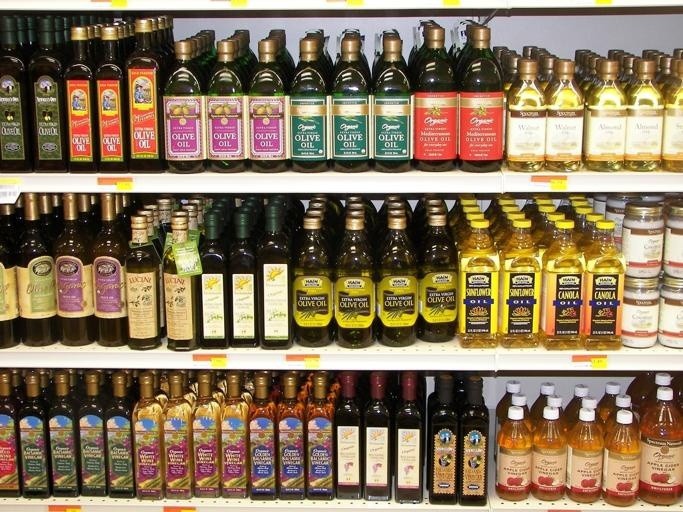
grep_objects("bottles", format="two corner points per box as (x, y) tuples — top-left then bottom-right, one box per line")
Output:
(1, 368), (683, 509)
(1, 194), (622, 352)
(0, 13), (682, 171)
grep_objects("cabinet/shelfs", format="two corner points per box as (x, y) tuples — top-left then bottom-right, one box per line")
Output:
(1, 10), (681, 512)
